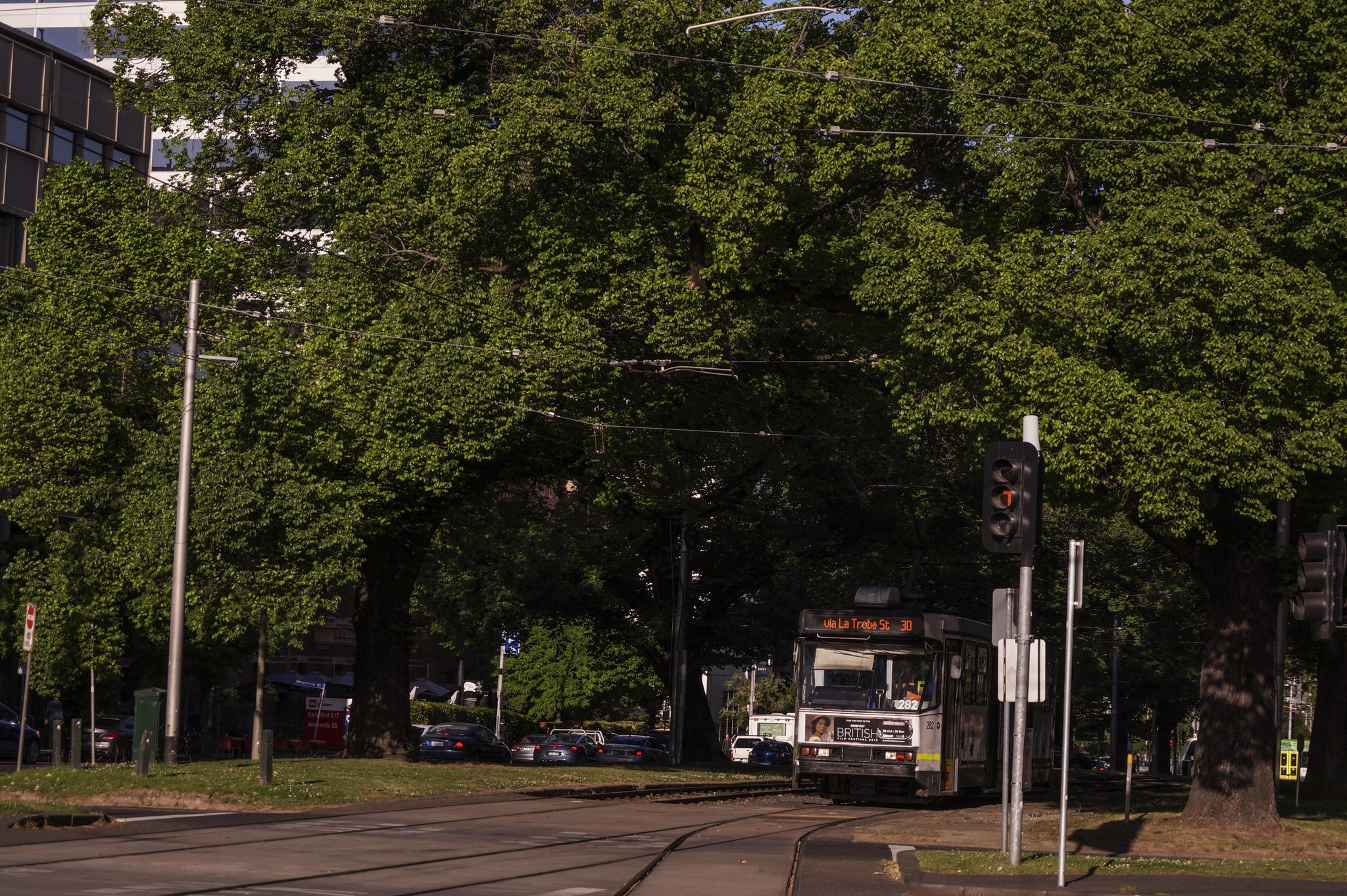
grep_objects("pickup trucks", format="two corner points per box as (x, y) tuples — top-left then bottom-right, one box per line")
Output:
(549, 729), (609, 746)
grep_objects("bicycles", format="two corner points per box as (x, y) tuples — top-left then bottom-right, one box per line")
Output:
(180, 728), (204, 761)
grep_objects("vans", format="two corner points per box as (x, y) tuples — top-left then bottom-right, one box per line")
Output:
(1176, 736), (1198, 778)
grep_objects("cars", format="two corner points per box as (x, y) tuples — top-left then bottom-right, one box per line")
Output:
(411, 724), (435, 736)
(533, 733), (602, 767)
(418, 722), (512, 764)
(1133, 760), (1150, 773)
(596, 734), (670, 765)
(81, 714), (136, 764)
(511, 734), (551, 765)
(0, 702), (42, 764)
(748, 739), (793, 765)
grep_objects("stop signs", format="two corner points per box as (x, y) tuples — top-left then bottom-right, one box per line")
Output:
(27, 605), (35, 629)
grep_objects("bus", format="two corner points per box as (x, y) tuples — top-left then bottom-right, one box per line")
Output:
(1280, 739), (1310, 782)
(749, 713), (796, 748)
(792, 583), (1059, 807)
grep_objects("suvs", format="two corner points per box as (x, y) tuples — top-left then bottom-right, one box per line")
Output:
(730, 734), (777, 763)
(1069, 752), (1110, 771)
(646, 728), (670, 748)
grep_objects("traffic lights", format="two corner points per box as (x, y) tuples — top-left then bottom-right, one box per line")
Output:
(982, 439), (1039, 554)
(1288, 529), (1336, 621)
